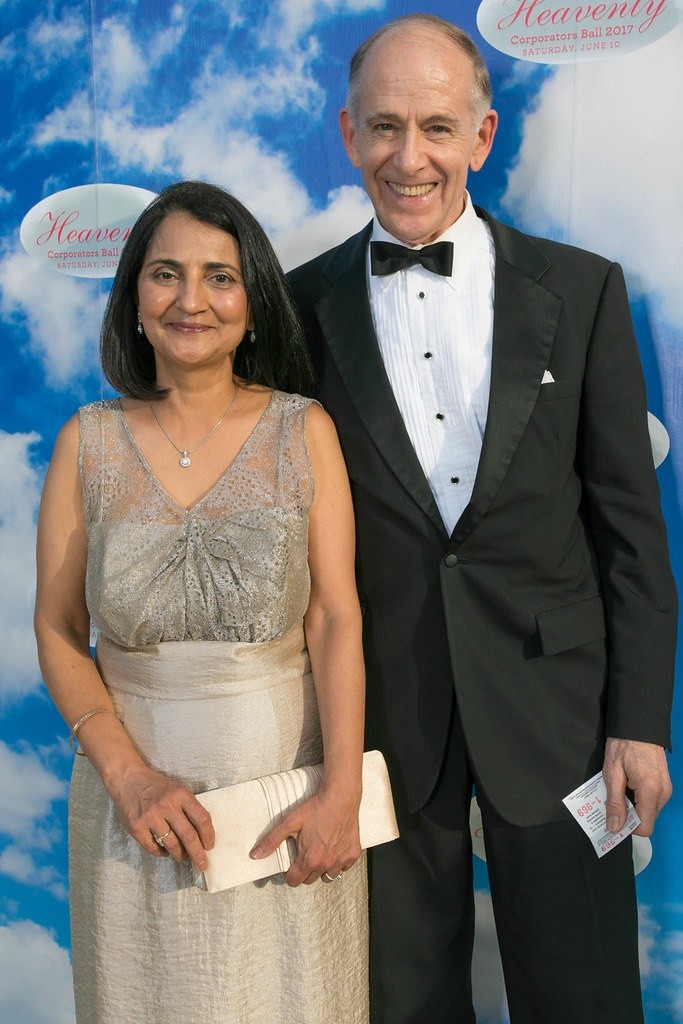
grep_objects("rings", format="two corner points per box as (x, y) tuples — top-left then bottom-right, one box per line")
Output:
(153, 828), (172, 847)
(325, 871), (344, 882)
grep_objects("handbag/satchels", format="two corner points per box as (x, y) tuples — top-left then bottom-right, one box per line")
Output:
(192, 750), (400, 894)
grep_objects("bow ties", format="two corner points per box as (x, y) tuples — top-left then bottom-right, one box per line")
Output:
(370, 241), (454, 277)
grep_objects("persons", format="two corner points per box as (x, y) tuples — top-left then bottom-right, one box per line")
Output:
(284, 14), (678, 1024)
(34, 181), (369, 1024)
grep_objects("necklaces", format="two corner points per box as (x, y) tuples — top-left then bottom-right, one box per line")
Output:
(149, 383), (238, 467)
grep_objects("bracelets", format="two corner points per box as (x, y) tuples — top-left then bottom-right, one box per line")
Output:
(69, 708), (125, 757)
